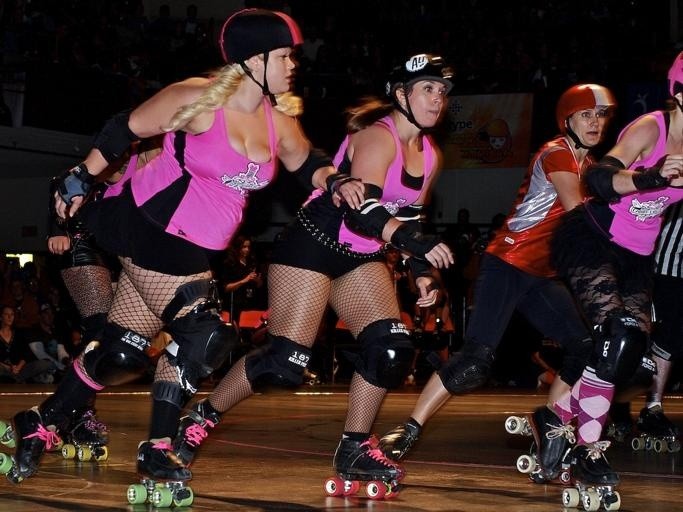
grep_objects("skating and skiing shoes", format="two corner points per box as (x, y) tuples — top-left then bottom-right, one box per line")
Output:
(127, 438), (194, 508)
(54, 406), (111, 462)
(505, 404), (576, 481)
(323, 434), (406, 500)
(0, 404), (62, 484)
(370, 422), (424, 459)
(172, 398), (222, 468)
(562, 441), (622, 511)
(631, 402), (682, 453)
(602, 423), (629, 447)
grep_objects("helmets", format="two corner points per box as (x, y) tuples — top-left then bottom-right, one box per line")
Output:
(665, 51), (683, 94)
(556, 84), (616, 135)
(220, 7), (303, 64)
(384, 53), (454, 95)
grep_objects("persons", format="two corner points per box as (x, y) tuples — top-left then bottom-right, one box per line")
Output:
(443, 205), (481, 258)
(524, 46), (683, 489)
(221, 238), (265, 321)
(0, 269), (80, 387)
(466, 214), (518, 389)
(10, 6), (367, 489)
(383, 247), (458, 386)
(609, 203), (683, 441)
(476, 119), (517, 167)
(38, 133), (171, 448)
(375, 78), (618, 463)
(169, 52), (458, 484)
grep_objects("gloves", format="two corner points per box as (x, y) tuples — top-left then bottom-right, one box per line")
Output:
(47, 203), (72, 238)
(52, 162), (97, 205)
(391, 223), (443, 259)
(326, 173), (354, 203)
(407, 256), (442, 295)
(632, 155), (672, 191)
